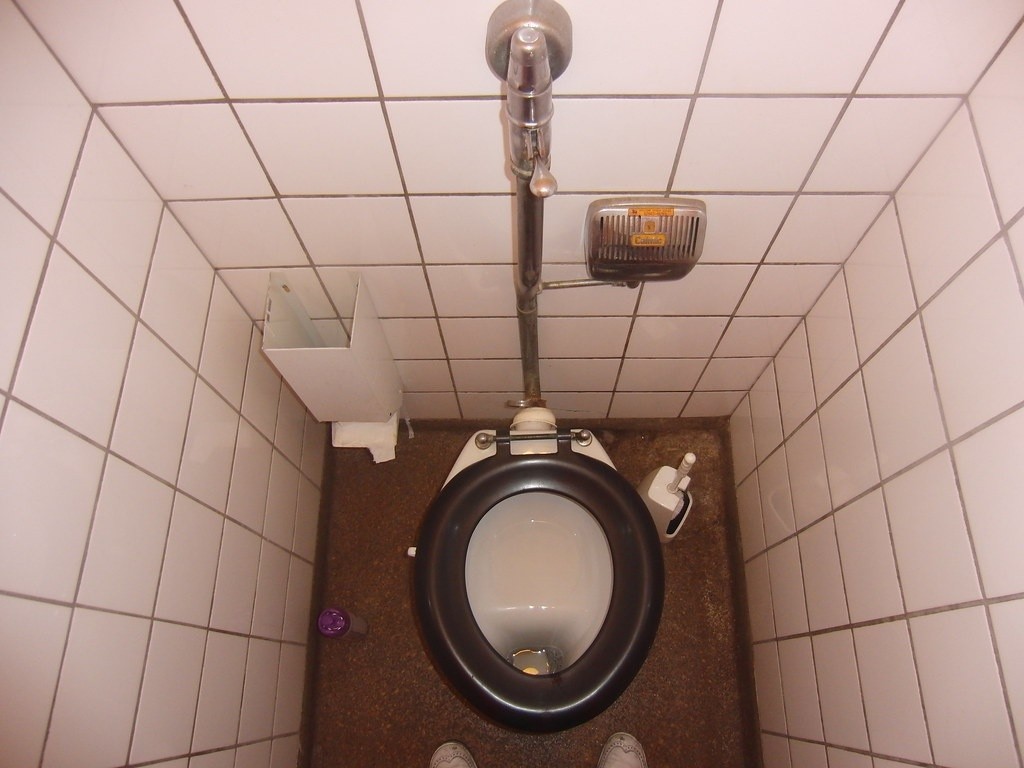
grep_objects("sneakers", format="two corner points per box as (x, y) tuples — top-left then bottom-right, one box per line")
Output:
(429, 742), (478, 768)
(595, 732), (648, 768)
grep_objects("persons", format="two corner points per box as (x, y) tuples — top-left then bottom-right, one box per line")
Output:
(428, 731), (647, 768)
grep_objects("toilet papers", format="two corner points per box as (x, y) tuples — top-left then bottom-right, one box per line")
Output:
(332, 412), (399, 464)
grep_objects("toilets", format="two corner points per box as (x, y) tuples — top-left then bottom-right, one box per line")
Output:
(410, 406), (668, 729)
